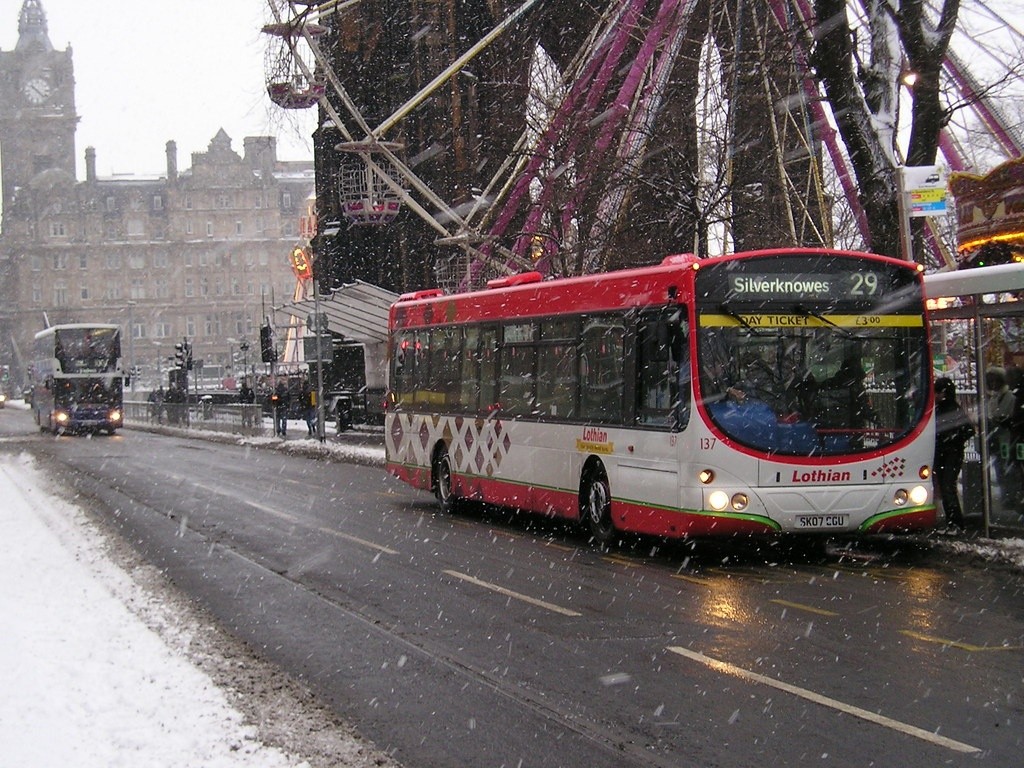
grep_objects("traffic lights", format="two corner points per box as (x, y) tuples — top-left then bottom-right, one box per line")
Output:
(175, 343), (185, 368)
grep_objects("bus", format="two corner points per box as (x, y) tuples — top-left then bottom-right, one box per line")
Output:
(31, 323), (130, 436)
(382, 248), (937, 552)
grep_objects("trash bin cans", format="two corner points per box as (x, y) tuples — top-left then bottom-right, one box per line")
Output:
(201, 395), (214, 420)
(332, 395), (353, 431)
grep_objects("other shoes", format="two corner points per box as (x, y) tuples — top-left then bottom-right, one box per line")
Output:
(936, 514), (963, 526)
(309, 431), (311, 435)
(313, 429), (315, 432)
(282, 431), (286, 435)
(277, 429), (281, 435)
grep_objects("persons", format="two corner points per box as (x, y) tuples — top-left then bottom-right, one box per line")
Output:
(684, 349), (744, 405)
(145, 384), (188, 425)
(982, 364), (1024, 515)
(299, 380), (317, 436)
(932, 377), (975, 534)
(271, 378), (289, 436)
(238, 382), (255, 427)
